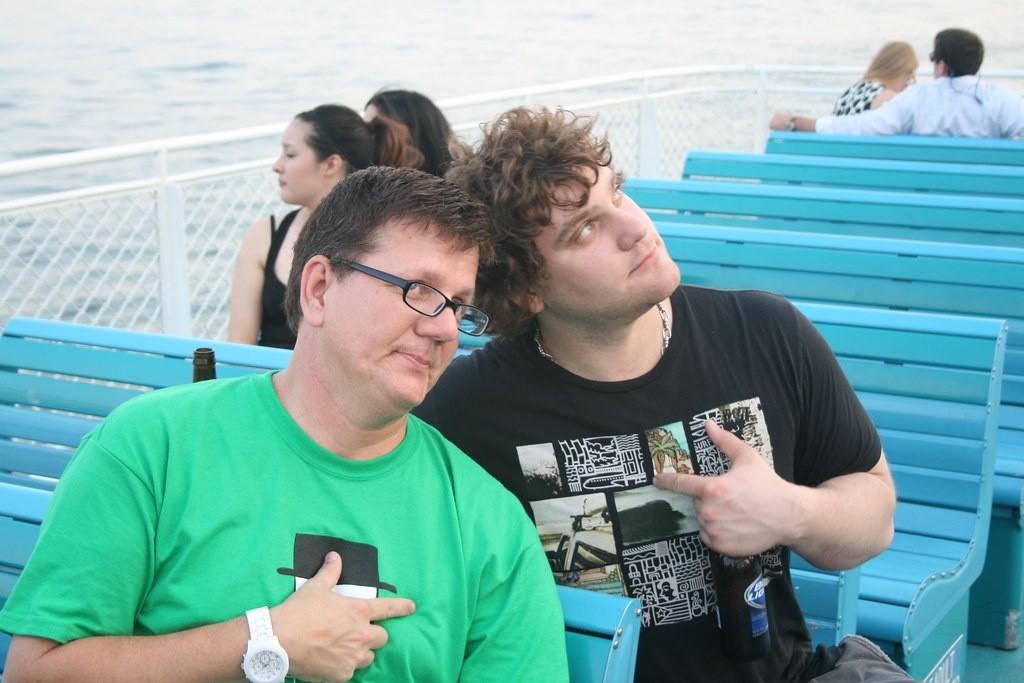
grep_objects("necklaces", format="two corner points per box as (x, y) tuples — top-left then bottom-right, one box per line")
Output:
(533, 301), (673, 364)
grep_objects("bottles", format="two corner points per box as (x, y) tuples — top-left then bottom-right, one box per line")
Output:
(710, 546), (771, 663)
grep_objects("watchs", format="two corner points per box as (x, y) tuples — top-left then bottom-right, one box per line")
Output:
(241, 605), (290, 683)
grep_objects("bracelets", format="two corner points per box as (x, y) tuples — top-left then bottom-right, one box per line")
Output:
(786, 118), (798, 131)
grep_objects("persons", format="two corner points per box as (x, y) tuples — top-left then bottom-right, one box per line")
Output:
(0, 166), (572, 683)
(409, 109), (921, 683)
(365, 91), (455, 180)
(833, 40), (920, 116)
(767, 27), (1023, 140)
(224, 105), (426, 351)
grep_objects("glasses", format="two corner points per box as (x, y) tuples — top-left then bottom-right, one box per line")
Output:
(330, 256), (491, 338)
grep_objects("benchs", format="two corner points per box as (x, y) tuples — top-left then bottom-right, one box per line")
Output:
(1, 130), (1024, 683)
